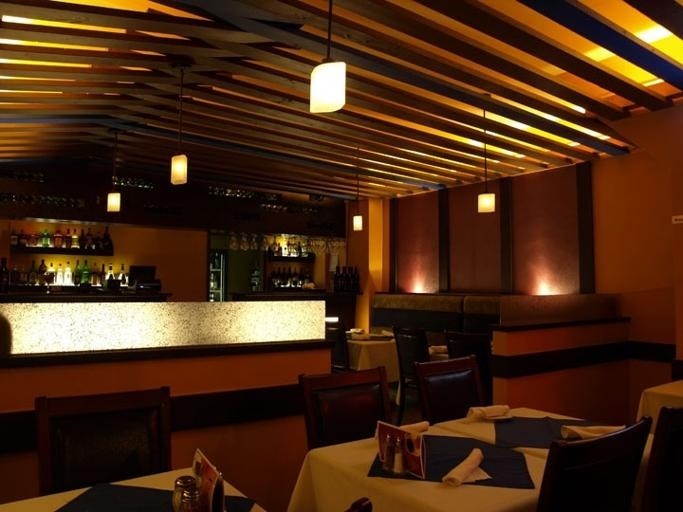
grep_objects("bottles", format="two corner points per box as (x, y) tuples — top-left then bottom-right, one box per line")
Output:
(268, 236), (282, 256)
(172, 475), (197, 512)
(250, 267), (263, 292)
(272, 267), (303, 287)
(178, 488), (201, 511)
(209, 252), (221, 302)
(0, 257), (129, 288)
(9, 225), (111, 249)
(333, 266), (360, 293)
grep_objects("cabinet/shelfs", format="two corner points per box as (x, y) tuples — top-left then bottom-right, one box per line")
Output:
(0, 244), (139, 297)
(227, 234), (316, 297)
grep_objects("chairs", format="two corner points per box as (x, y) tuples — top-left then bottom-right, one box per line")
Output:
(36, 384), (170, 496)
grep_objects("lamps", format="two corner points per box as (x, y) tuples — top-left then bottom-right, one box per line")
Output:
(477, 105), (496, 212)
(108, 130), (122, 212)
(171, 63), (188, 185)
(352, 145), (363, 232)
(310, 0), (347, 114)
